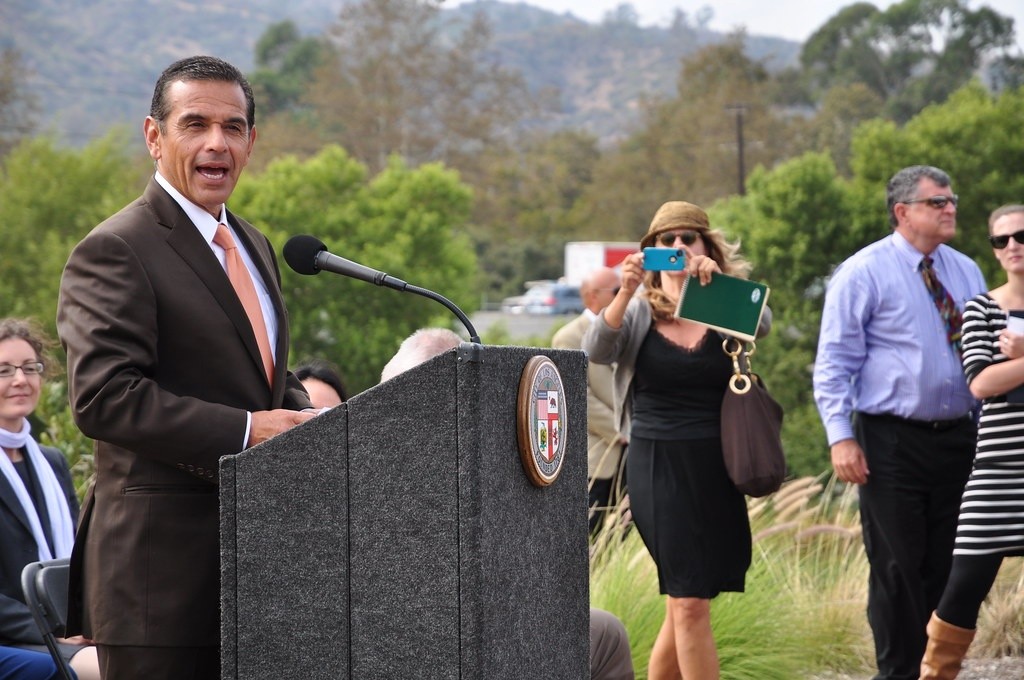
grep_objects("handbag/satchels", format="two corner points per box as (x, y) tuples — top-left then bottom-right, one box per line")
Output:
(720, 334), (786, 498)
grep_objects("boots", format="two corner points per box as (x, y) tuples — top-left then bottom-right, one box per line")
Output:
(917, 610), (978, 680)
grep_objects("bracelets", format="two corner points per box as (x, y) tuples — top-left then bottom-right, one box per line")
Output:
(619, 287), (636, 294)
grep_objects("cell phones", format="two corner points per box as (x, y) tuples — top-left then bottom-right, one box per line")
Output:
(642, 247), (687, 271)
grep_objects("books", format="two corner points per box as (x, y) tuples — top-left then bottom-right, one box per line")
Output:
(673, 269), (771, 342)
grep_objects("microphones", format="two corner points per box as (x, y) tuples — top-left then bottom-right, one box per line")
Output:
(283, 235), (407, 291)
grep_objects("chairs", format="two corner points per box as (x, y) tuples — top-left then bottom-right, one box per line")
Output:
(21, 557), (72, 680)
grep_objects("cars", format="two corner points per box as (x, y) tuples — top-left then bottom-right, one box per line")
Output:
(502, 282), (585, 316)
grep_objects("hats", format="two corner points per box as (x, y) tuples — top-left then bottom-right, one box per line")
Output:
(640, 200), (711, 251)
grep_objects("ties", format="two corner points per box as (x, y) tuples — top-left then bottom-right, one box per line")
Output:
(919, 255), (963, 365)
(212, 223), (274, 394)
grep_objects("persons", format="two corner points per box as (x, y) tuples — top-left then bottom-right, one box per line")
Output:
(0, 314), (101, 680)
(292, 357), (348, 413)
(53, 56), (324, 680)
(379, 328), (634, 680)
(580, 200), (773, 680)
(917, 203), (1024, 680)
(552, 265), (633, 548)
(812, 165), (990, 680)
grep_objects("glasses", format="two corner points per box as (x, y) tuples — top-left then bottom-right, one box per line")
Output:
(0, 361), (46, 378)
(595, 285), (621, 296)
(988, 230), (1024, 250)
(655, 231), (702, 247)
(903, 193), (959, 210)
